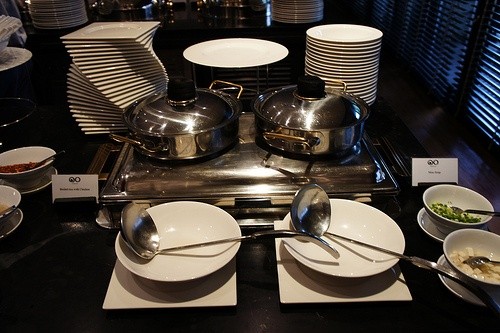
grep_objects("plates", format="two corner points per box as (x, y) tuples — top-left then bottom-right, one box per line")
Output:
(182, 38), (289, 68)
(270, 0), (325, 24)
(102, 258), (237, 309)
(305, 24), (384, 106)
(437, 254), (500, 307)
(0, 166), (58, 195)
(0, 208), (24, 240)
(60, 21), (170, 134)
(28, 0), (88, 29)
(274, 220), (413, 303)
(417, 207), (488, 242)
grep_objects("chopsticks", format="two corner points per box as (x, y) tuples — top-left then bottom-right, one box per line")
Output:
(380, 135), (411, 177)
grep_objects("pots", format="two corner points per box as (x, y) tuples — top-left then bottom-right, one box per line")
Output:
(250, 74), (371, 155)
(109, 76), (244, 160)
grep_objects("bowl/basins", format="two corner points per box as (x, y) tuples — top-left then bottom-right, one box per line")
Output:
(0, 147), (56, 189)
(0, 185), (22, 231)
(281, 198), (405, 277)
(114, 201), (242, 282)
(0, 98), (37, 149)
(443, 228), (500, 285)
(422, 184), (495, 235)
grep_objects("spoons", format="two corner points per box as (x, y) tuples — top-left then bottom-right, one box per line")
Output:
(450, 206), (500, 217)
(465, 256), (500, 270)
